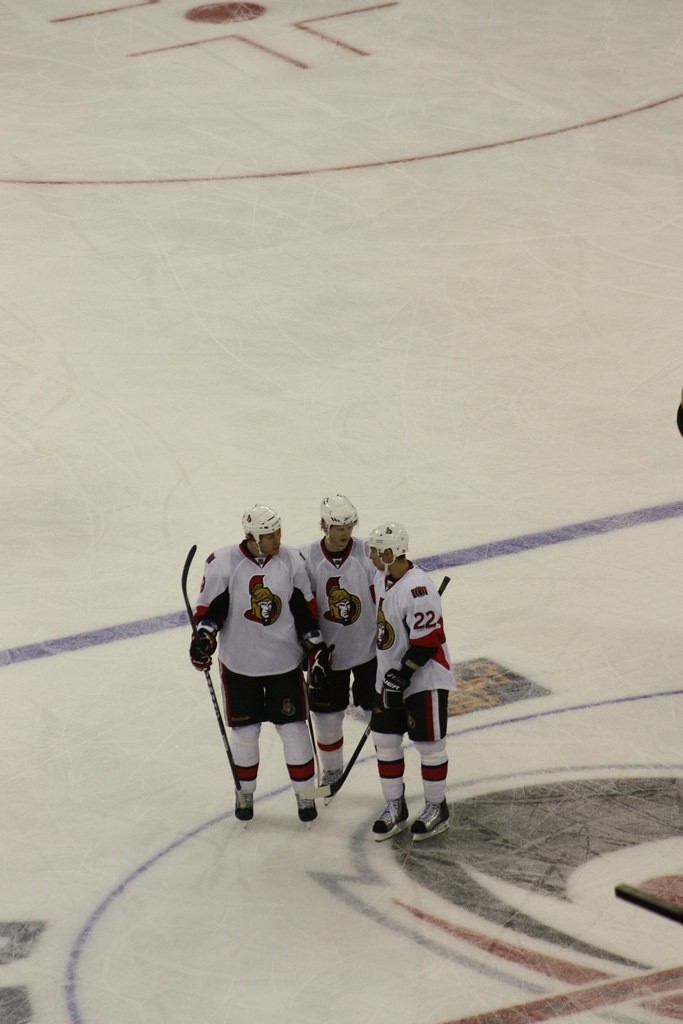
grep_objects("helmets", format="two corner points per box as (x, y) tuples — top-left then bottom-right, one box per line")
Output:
(319, 494), (359, 531)
(369, 522), (408, 565)
(242, 504), (281, 542)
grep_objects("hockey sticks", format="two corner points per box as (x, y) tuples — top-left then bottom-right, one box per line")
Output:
(295, 573), (453, 804)
(177, 540), (250, 814)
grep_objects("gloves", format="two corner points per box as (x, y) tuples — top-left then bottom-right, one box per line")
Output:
(309, 641), (335, 688)
(379, 668), (410, 714)
(189, 628), (217, 671)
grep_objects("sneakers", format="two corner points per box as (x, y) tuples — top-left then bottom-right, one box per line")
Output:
(411, 798), (450, 841)
(372, 783), (409, 841)
(234, 788), (254, 830)
(295, 792), (318, 830)
(320, 764), (344, 806)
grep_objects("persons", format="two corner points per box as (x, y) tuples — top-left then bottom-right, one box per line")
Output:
(367, 522), (457, 835)
(189, 505), (330, 822)
(298, 494), (379, 788)
